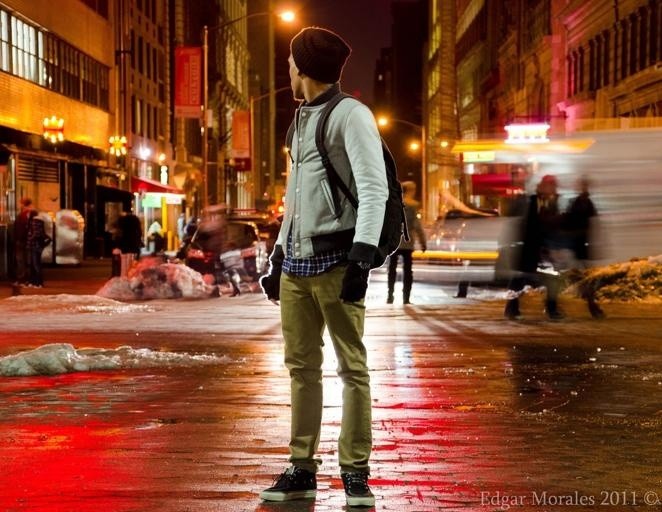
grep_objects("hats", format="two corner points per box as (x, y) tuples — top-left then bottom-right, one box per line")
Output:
(21, 198), (38, 216)
(533, 172), (596, 196)
(290, 24), (353, 85)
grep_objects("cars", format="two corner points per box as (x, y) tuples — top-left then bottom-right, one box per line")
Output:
(186, 215), (270, 282)
(424, 209), (499, 277)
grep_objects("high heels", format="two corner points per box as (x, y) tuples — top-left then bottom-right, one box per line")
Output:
(206, 285), (242, 299)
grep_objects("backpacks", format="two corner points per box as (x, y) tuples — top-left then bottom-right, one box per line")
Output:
(285, 92), (402, 267)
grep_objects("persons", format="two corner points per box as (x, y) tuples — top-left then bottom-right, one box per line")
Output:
(25, 209), (52, 289)
(11, 197), (34, 287)
(543, 175), (605, 319)
(386, 181), (427, 305)
(116, 200), (142, 276)
(504, 176), (566, 322)
(258, 29), (412, 505)
(103, 191), (137, 276)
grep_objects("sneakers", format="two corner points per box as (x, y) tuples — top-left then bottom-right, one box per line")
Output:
(341, 472), (378, 509)
(12, 282), (47, 289)
(258, 466), (317, 502)
(504, 308), (609, 323)
(385, 296), (413, 307)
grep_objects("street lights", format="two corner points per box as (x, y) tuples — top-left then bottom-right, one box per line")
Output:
(203, 12), (292, 211)
(379, 119), (426, 224)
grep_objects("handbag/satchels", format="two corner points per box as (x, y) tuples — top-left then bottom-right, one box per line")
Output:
(506, 214), (569, 273)
(37, 233), (51, 248)
(217, 249), (245, 271)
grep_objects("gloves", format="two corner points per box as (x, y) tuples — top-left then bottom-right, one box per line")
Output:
(259, 245), (284, 302)
(339, 242), (377, 304)
